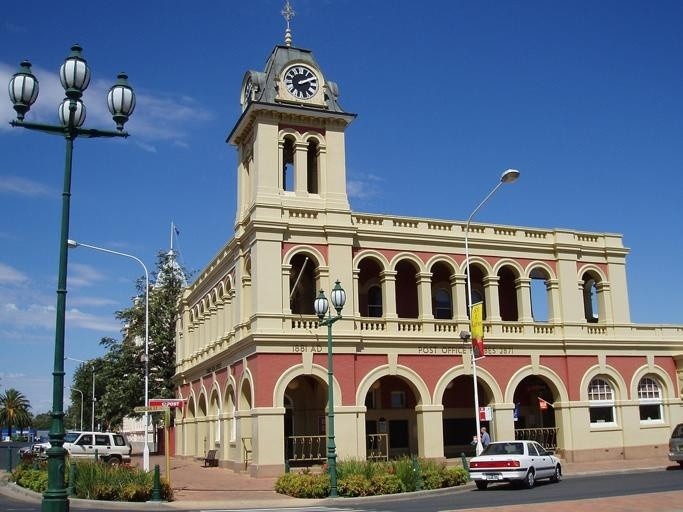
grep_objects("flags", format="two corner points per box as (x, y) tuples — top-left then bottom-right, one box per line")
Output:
(537, 397), (547, 409)
(513, 404), (519, 421)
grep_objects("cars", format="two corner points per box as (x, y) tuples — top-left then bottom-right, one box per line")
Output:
(668, 423), (683, 468)
(468, 439), (563, 490)
(17, 431), (133, 466)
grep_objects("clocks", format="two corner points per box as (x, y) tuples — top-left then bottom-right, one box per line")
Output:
(283, 65), (319, 99)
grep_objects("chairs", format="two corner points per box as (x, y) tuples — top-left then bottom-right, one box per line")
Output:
(203, 450), (216, 467)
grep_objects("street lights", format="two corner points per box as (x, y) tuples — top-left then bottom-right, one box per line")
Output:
(9, 42), (136, 511)
(459, 168), (520, 459)
(64, 357), (95, 431)
(64, 386), (84, 431)
(68, 239), (150, 473)
(313, 279), (346, 496)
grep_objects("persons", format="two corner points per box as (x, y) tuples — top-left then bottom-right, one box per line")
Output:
(480, 427), (490, 448)
